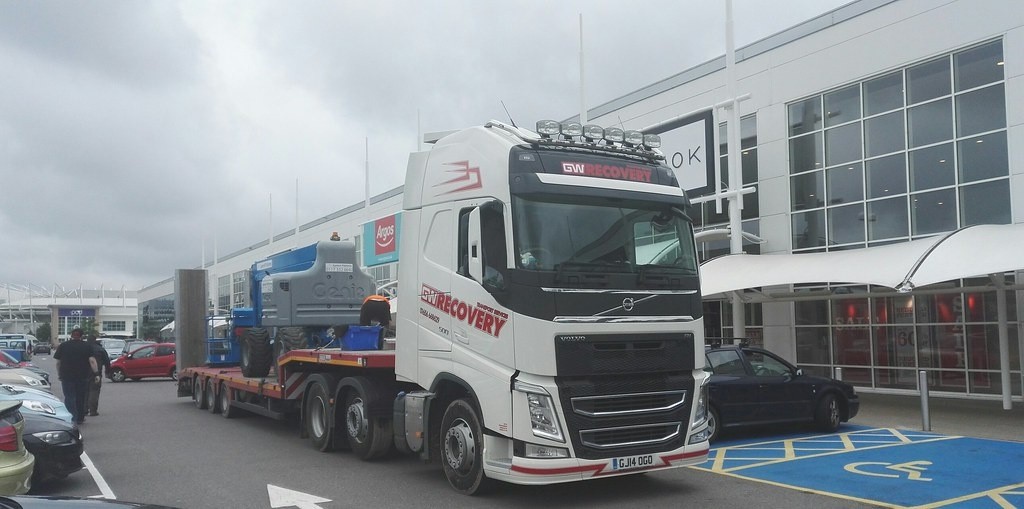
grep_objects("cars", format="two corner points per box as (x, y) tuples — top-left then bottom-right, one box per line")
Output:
(703, 335), (860, 447)
(0, 398), (35, 496)
(33, 342), (51, 356)
(0, 334), (85, 490)
(105, 342), (178, 382)
(95, 337), (157, 361)
(0, 494), (182, 509)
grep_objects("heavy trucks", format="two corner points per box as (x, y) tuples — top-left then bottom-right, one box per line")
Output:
(171, 118), (714, 495)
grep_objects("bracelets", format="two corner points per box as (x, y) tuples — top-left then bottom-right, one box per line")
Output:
(93, 371), (99, 376)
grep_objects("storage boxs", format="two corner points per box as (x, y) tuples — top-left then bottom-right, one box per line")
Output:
(333, 325), (382, 351)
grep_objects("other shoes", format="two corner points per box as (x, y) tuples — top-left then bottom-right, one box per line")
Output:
(90, 413), (98, 416)
(71, 416), (82, 424)
(82, 411), (88, 415)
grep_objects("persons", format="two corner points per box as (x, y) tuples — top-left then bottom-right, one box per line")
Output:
(83, 335), (111, 416)
(53, 329), (101, 426)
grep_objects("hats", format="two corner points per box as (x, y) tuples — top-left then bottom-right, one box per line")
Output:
(71, 329), (82, 338)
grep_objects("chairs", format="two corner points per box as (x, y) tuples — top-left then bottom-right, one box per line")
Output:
(732, 358), (745, 373)
(718, 358), (733, 374)
